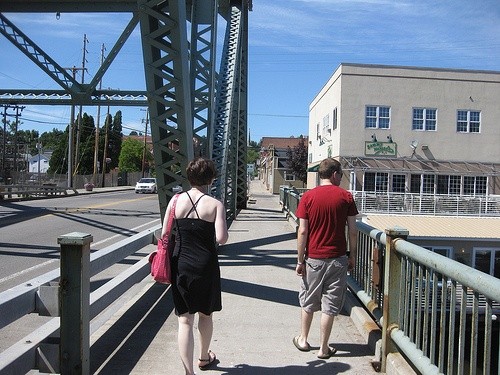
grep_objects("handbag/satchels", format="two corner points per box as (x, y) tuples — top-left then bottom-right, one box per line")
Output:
(148, 194), (179, 285)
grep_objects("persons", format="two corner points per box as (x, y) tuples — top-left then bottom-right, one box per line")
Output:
(161, 157), (228, 375)
(293, 158), (359, 359)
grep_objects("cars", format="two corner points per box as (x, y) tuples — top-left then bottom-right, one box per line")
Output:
(135, 178), (158, 194)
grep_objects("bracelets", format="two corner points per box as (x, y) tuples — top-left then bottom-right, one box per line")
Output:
(297, 262), (305, 265)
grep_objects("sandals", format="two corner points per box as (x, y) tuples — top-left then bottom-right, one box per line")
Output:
(198, 352), (216, 370)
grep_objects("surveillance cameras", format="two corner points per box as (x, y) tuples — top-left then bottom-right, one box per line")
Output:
(387, 134), (391, 139)
(371, 133), (375, 138)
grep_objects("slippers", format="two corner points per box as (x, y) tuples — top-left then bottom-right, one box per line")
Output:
(317, 347), (336, 359)
(292, 334), (310, 351)
